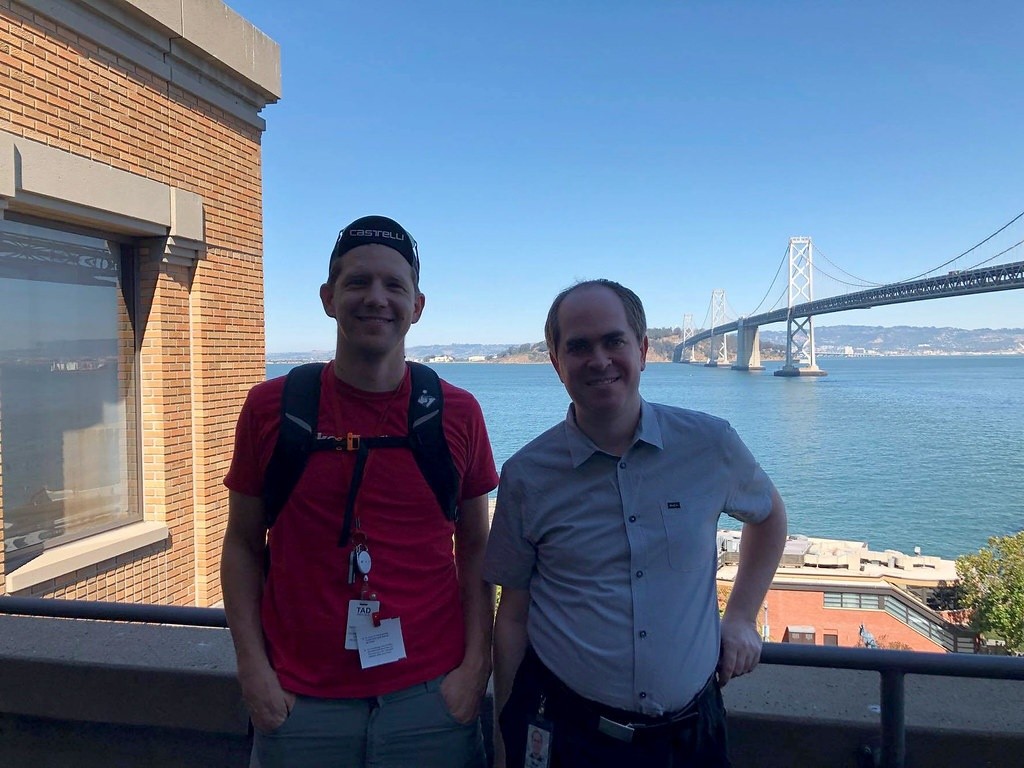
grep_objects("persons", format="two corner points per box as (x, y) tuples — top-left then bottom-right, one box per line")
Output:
(221, 215), (500, 768)
(482, 279), (788, 768)
(528, 729), (544, 763)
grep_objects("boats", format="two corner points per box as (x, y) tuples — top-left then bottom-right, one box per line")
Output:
(44, 482), (122, 501)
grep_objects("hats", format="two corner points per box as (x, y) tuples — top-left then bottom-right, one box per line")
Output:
(330, 215), (419, 276)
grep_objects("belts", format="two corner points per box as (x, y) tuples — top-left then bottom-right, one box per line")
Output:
(527, 647), (716, 742)
